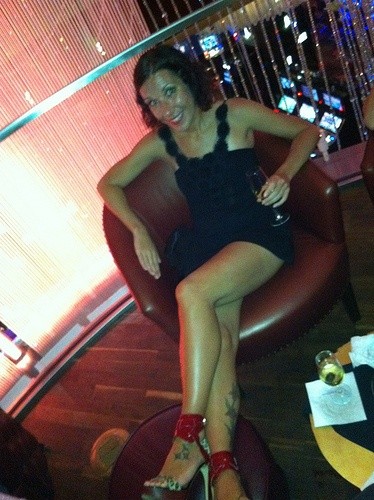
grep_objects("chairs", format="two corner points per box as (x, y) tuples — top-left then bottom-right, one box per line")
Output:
(103, 132), (361, 400)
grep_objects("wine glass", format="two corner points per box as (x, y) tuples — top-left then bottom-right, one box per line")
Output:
(316, 350), (353, 405)
(245, 166), (291, 227)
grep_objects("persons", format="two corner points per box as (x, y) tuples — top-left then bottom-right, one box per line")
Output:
(97, 43), (319, 500)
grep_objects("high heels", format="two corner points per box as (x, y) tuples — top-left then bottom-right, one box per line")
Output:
(142, 413), (250, 499)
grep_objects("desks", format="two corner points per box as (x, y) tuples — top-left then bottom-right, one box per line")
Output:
(308, 332), (374, 492)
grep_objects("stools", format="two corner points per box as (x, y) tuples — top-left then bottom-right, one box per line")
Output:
(107, 404), (290, 500)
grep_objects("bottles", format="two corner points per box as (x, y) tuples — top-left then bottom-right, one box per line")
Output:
(0, 321), (42, 378)
